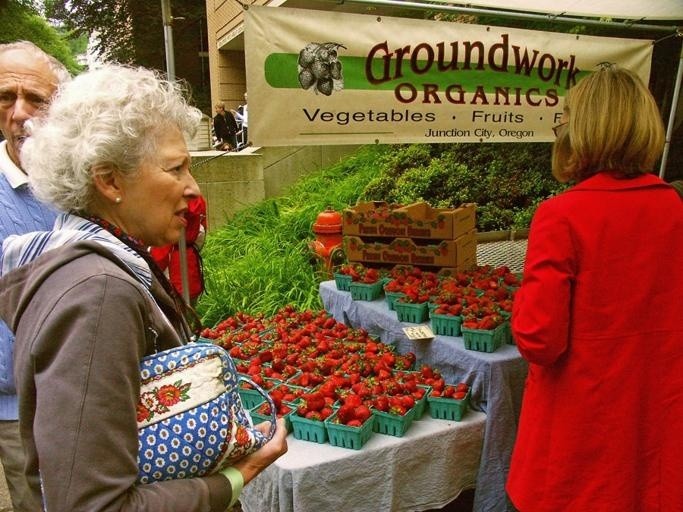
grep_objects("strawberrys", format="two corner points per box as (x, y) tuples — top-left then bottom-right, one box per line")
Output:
(333, 262), (522, 353)
(199, 304), (468, 427)
(346, 216), (448, 257)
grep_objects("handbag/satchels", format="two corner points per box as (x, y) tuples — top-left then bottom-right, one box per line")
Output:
(38, 343), (277, 512)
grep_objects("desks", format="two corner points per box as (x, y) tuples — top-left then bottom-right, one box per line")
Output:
(240, 236), (529, 510)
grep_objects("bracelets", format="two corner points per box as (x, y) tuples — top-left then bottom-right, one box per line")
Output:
(220, 467), (246, 510)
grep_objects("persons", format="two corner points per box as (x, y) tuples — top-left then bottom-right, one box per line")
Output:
(504, 66), (683, 512)
(150, 195), (208, 310)
(214, 101), (237, 148)
(230, 92), (248, 141)
(0, 41), (73, 512)
(0, 67), (288, 512)
